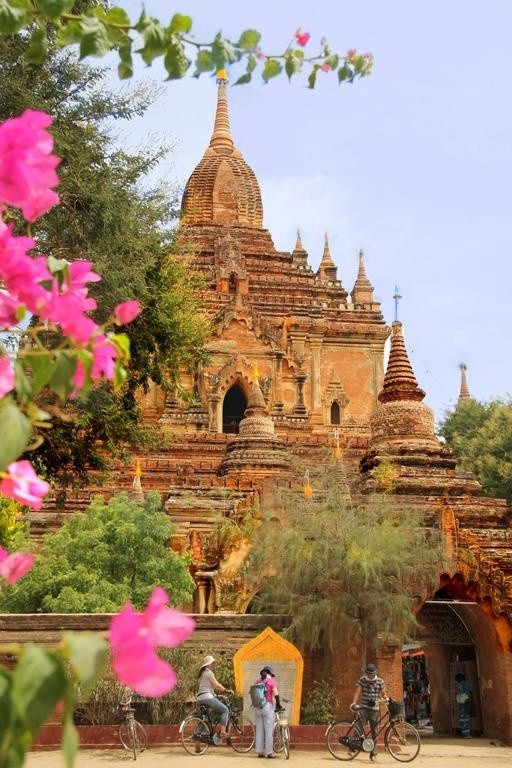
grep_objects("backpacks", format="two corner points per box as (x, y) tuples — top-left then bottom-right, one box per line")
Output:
(250, 680), (269, 709)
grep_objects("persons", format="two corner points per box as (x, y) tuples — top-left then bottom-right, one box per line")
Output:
(348, 664), (389, 761)
(455, 673), (473, 739)
(195, 656), (234, 753)
(255, 666), (282, 758)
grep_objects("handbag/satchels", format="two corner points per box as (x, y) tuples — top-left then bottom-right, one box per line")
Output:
(465, 703), (476, 716)
(456, 693), (469, 704)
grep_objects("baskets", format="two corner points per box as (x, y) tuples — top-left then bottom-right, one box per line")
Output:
(231, 693), (244, 713)
(387, 701), (406, 718)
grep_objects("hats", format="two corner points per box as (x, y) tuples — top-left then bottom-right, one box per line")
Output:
(365, 664), (378, 675)
(265, 666), (276, 677)
(201, 656), (215, 668)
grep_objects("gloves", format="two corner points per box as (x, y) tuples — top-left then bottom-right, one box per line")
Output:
(225, 689), (234, 695)
(274, 695), (283, 709)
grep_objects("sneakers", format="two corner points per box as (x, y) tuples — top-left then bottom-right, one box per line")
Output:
(219, 733), (230, 737)
(195, 748), (203, 753)
(258, 751), (280, 758)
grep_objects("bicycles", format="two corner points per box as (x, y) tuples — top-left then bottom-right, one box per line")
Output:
(252, 696), (295, 760)
(117, 692), (150, 761)
(176, 686), (257, 755)
(326, 699), (422, 763)
